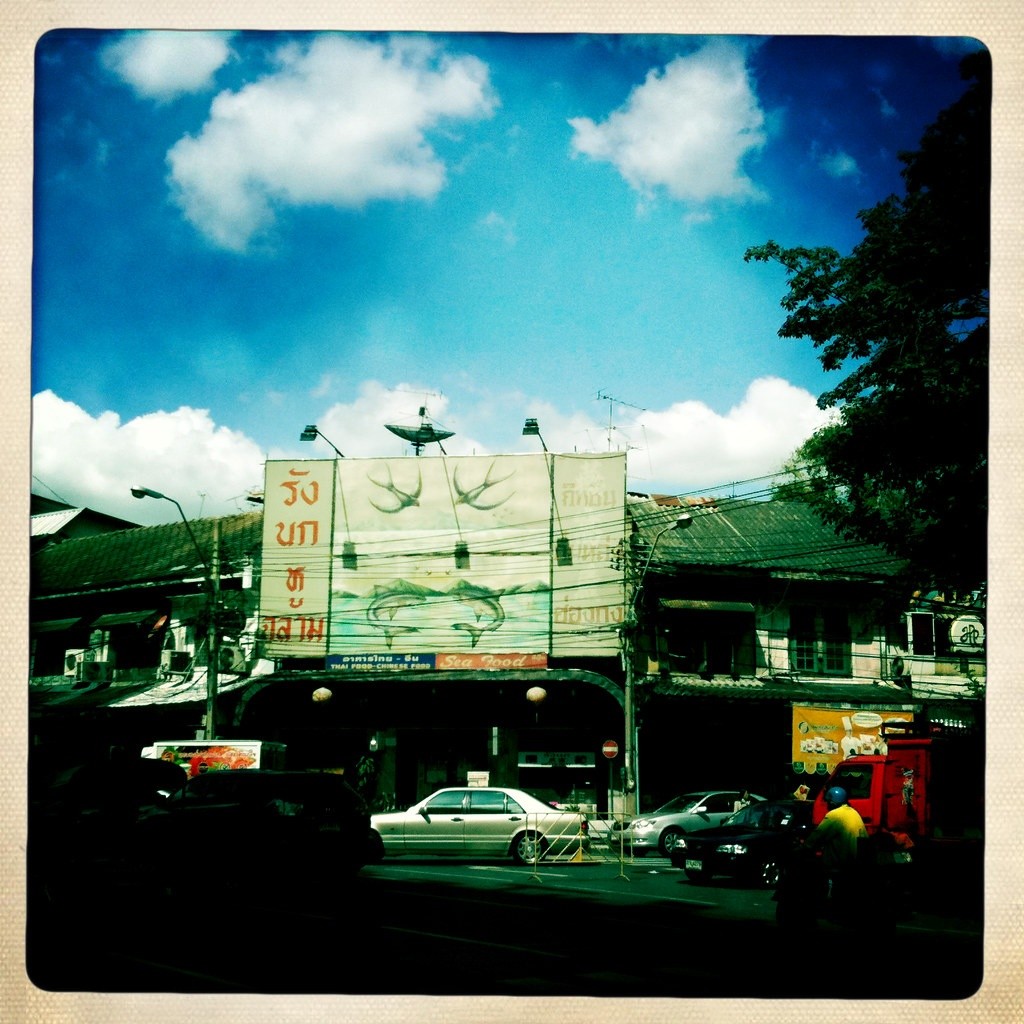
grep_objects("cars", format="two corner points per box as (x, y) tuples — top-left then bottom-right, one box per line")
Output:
(620, 790), (796, 859)
(671, 798), (816, 891)
(131, 767), (374, 894)
(368, 785), (592, 867)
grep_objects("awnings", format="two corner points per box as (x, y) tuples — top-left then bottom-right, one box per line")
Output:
(28, 673), (271, 709)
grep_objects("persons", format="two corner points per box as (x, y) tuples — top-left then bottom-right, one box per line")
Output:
(734, 790), (752, 823)
(800, 785), (870, 937)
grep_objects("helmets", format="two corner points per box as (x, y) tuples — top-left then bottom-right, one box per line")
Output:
(824, 786), (848, 807)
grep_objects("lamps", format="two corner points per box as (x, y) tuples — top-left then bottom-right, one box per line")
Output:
(522, 419), (540, 436)
(299, 424), (317, 440)
(418, 422), (433, 444)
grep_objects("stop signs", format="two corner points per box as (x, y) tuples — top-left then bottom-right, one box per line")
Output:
(602, 738), (619, 760)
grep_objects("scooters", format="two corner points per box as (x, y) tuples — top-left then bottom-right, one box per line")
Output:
(772, 841), (899, 947)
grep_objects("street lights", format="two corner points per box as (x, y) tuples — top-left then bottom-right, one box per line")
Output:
(620, 513), (695, 795)
(129, 484), (223, 741)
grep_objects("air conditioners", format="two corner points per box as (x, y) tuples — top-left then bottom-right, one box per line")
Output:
(161, 648), (191, 672)
(65, 647), (96, 677)
(218, 645), (248, 672)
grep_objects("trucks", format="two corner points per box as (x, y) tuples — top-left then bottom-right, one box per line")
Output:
(810, 738), (986, 859)
